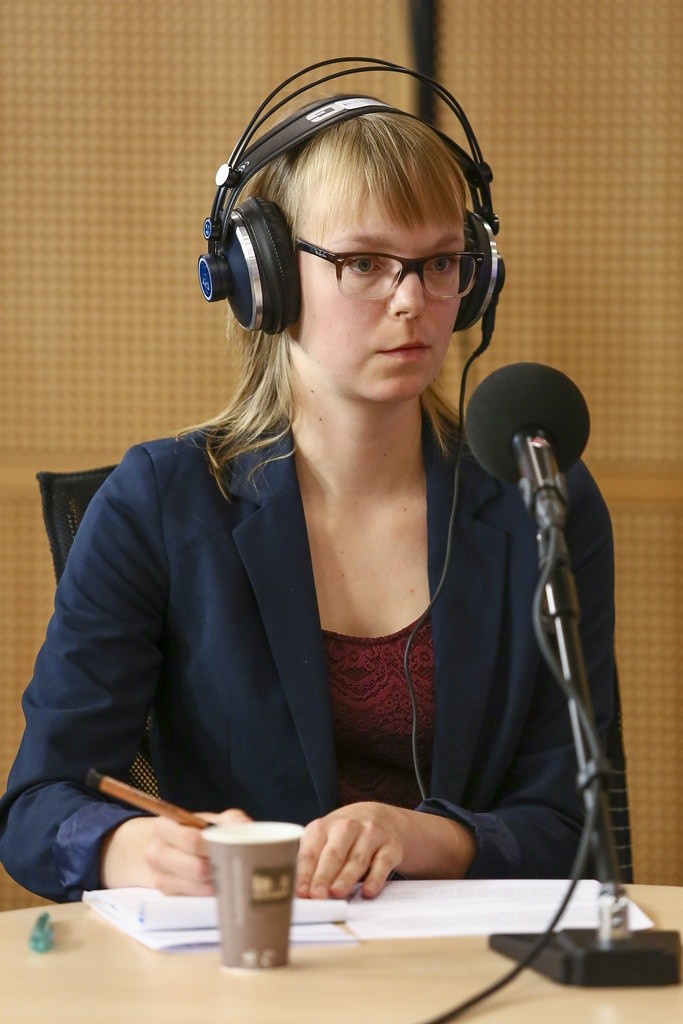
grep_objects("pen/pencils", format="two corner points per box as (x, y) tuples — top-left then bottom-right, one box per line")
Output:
(28, 912), (52, 953)
(85, 769), (215, 829)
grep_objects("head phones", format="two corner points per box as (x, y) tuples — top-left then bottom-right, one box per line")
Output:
(198, 57), (503, 336)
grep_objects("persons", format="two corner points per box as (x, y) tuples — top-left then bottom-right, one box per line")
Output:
(1, 94), (633, 898)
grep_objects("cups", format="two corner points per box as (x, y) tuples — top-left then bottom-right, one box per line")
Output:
(201, 822), (304, 973)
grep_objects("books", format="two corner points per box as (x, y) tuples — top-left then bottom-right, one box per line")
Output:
(80, 888), (353, 950)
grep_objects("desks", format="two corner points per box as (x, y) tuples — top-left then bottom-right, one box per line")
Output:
(0, 879), (683, 1024)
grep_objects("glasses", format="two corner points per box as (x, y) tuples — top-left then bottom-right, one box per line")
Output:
(296, 237), (486, 301)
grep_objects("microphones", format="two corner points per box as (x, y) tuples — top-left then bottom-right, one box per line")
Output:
(466, 359), (683, 989)
